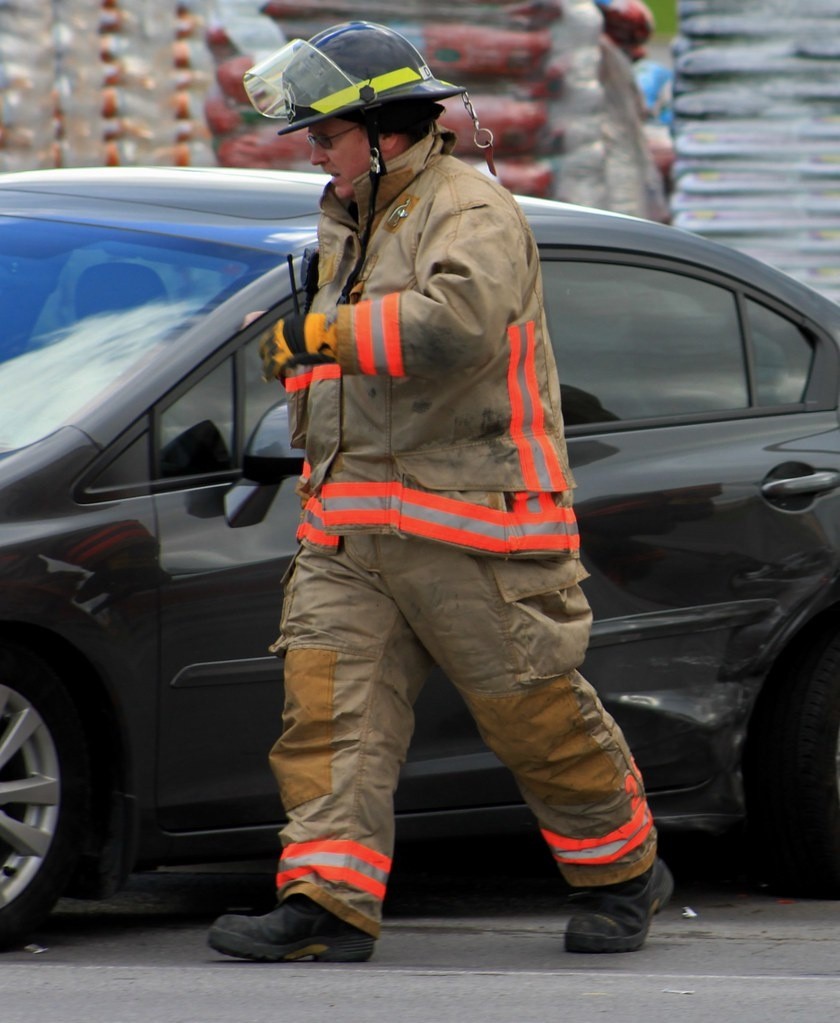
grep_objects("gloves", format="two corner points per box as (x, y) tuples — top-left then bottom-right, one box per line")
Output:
(258, 313), (338, 384)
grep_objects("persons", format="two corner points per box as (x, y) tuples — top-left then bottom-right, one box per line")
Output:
(72, 267), (166, 320)
(207, 19), (673, 960)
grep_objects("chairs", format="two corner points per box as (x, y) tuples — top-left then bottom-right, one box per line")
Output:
(74, 263), (172, 346)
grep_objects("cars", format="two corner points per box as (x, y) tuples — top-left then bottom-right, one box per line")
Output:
(1, 164), (840, 948)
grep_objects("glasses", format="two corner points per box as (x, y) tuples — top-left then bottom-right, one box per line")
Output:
(305, 120), (360, 150)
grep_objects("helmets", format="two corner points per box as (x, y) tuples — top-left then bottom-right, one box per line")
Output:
(242, 20), (466, 135)
(603, 0), (653, 57)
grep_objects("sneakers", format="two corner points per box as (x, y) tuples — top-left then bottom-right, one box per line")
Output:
(564, 857), (673, 951)
(208, 894), (374, 964)
